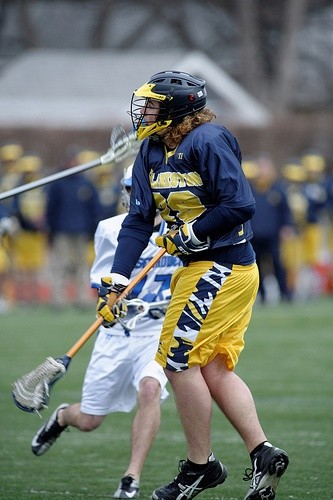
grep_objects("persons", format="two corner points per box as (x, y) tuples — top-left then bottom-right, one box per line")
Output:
(0, 144), (333, 305)
(107, 70), (289, 500)
(31, 163), (182, 497)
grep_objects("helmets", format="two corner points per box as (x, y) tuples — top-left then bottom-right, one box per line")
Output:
(135, 70), (207, 122)
(121, 163), (134, 213)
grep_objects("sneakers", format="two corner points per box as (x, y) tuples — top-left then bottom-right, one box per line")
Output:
(152, 452), (228, 500)
(242, 441), (289, 500)
(114, 474), (139, 498)
(30, 404), (70, 456)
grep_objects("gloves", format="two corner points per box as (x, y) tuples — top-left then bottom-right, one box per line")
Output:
(155, 223), (202, 258)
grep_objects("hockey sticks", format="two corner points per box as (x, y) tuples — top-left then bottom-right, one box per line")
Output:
(13, 248), (166, 413)
(112, 298), (173, 331)
(0, 123), (144, 203)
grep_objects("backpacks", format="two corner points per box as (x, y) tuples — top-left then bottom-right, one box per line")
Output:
(97, 276), (128, 328)
